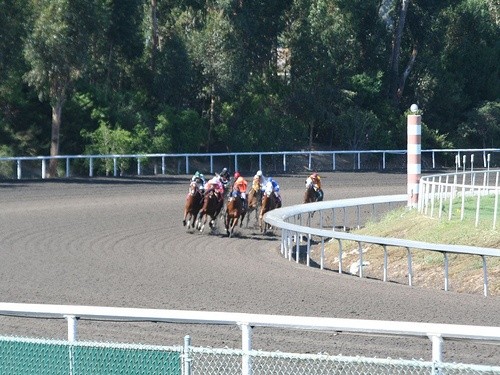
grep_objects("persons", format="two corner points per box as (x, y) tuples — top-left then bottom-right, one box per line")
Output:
(251, 169), (280, 203)
(230, 172), (248, 206)
(188, 168), (231, 198)
(306, 173), (322, 201)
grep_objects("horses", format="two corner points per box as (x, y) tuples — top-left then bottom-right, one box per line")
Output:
(297, 176), (324, 220)
(181, 175), (282, 238)
